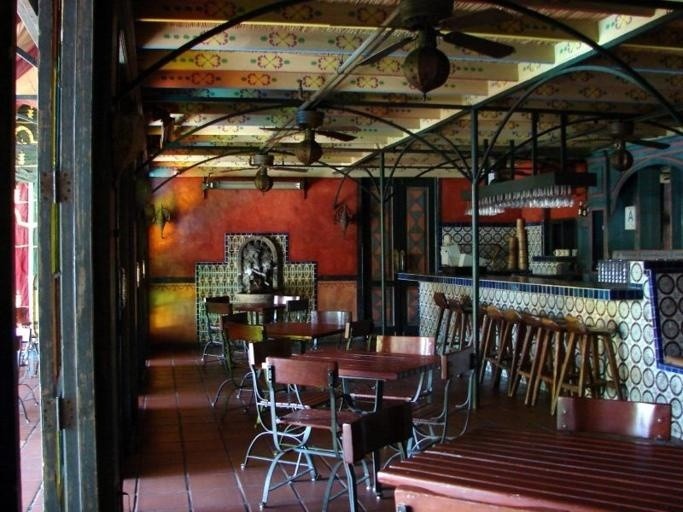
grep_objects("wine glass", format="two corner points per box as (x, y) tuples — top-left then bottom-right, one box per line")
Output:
(464, 185), (574, 217)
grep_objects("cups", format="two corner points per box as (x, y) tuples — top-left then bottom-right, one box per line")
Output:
(553, 249), (577, 257)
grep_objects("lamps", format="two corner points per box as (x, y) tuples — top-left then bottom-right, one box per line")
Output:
(400, 1), (451, 102)
(294, 109), (323, 167)
(335, 203), (357, 239)
(250, 151), (276, 193)
(606, 142), (635, 174)
(151, 199), (177, 242)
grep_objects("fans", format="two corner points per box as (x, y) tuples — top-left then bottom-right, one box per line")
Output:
(258, 109), (356, 142)
(574, 120), (671, 154)
(220, 149), (309, 174)
(347, 0), (520, 69)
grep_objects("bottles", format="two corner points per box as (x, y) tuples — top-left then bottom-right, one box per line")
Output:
(505, 163), (511, 180)
(488, 170), (496, 186)
(311, 305), (318, 325)
(597, 259), (629, 284)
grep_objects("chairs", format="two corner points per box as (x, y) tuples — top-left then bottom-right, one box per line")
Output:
(241, 340), (317, 482)
(556, 395), (672, 441)
(203, 287), (352, 425)
(387, 343), (479, 468)
(348, 333), (434, 430)
(262, 356), (366, 512)
(428, 292), (622, 415)
(342, 400), (413, 512)
